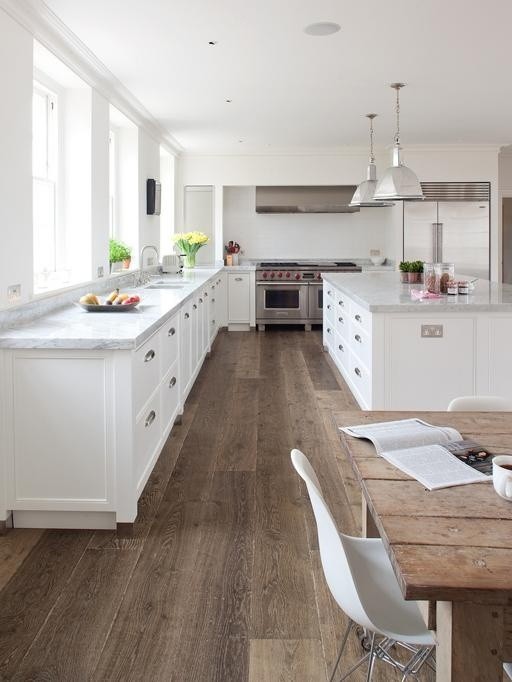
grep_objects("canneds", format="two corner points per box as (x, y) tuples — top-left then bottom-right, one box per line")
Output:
(446, 281), (458, 295)
(458, 280), (469, 295)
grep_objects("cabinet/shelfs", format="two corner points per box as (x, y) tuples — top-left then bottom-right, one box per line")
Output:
(323, 281), (512, 411)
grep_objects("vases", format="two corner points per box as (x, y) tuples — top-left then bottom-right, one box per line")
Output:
(185, 255), (196, 269)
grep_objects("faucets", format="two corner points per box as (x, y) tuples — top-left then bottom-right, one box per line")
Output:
(134, 244), (161, 287)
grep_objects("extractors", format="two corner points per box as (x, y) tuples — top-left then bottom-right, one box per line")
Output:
(255, 185), (360, 213)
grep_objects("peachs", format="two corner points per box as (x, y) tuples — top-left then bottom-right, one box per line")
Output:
(79, 293), (99, 305)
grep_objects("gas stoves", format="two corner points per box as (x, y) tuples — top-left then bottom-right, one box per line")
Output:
(256, 262), (363, 281)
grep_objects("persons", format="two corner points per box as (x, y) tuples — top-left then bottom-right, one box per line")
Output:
(452, 447), (489, 466)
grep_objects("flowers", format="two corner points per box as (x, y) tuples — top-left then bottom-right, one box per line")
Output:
(171, 229), (210, 267)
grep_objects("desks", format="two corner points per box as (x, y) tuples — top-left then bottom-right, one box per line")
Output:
(339, 412), (512, 681)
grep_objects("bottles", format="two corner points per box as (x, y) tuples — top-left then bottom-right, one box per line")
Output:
(227, 253), (239, 267)
(425, 263), (468, 296)
(448, 295), (468, 304)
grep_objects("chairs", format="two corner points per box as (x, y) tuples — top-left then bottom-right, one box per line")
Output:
(448, 394), (512, 412)
(290, 447), (436, 681)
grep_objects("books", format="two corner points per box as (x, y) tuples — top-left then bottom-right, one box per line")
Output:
(337, 419), (499, 494)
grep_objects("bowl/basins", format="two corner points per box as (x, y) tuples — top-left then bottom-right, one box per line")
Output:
(371, 257), (385, 265)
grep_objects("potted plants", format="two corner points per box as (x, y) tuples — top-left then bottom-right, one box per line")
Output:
(399, 260), (425, 284)
(110, 239), (131, 273)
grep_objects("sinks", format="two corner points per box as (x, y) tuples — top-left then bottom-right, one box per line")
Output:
(156, 281), (190, 285)
(143, 284), (184, 289)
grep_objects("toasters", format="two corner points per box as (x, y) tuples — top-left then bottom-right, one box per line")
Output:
(255, 281), (323, 331)
(163, 254), (186, 274)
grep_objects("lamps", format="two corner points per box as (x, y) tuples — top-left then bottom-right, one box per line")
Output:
(348, 81), (426, 209)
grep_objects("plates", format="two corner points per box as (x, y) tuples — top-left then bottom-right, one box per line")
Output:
(72, 297), (141, 311)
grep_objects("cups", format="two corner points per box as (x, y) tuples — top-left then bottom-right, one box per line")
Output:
(492, 454), (512, 501)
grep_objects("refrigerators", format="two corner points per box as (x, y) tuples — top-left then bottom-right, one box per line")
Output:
(401, 181), (490, 282)
